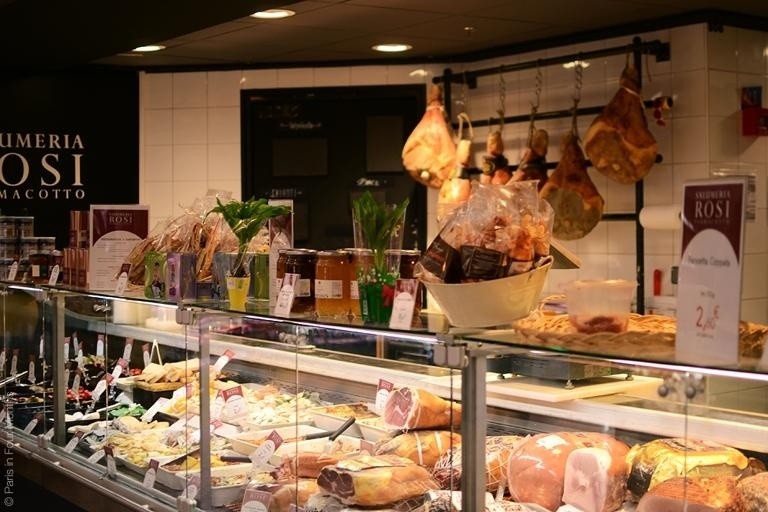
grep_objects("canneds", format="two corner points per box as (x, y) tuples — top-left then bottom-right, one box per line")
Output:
(276, 247), (422, 317)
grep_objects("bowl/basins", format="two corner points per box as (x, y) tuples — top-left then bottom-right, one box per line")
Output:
(418, 261), (553, 330)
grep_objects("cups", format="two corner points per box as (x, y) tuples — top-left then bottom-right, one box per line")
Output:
(226, 276), (252, 310)
